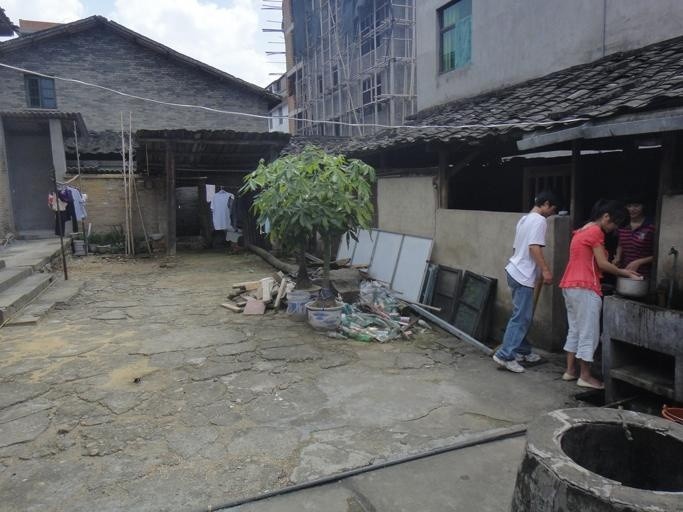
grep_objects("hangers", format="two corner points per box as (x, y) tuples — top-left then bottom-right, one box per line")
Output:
(218, 186), (227, 194)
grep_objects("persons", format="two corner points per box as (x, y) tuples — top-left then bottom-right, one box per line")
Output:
(559, 195), (656, 389)
(491, 190), (557, 374)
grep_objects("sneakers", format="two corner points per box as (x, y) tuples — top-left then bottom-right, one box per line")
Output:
(493, 352), (541, 373)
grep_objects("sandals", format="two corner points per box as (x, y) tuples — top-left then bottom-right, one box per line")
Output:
(562, 372), (605, 389)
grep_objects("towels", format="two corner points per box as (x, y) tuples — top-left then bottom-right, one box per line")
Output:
(206, 184), (215, 203)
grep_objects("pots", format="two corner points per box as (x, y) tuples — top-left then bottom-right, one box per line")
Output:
(616, 276), (650, 298)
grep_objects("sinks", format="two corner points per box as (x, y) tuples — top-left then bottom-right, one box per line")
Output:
(602, 289), (683, 357)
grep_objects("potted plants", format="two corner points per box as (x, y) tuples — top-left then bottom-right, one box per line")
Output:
(239, 142), (375, 331)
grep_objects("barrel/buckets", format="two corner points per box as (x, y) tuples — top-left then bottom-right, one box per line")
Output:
(286, 289), (311, 322)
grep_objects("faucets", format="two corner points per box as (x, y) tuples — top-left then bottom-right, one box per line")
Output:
(667, 245), (678, 256)
